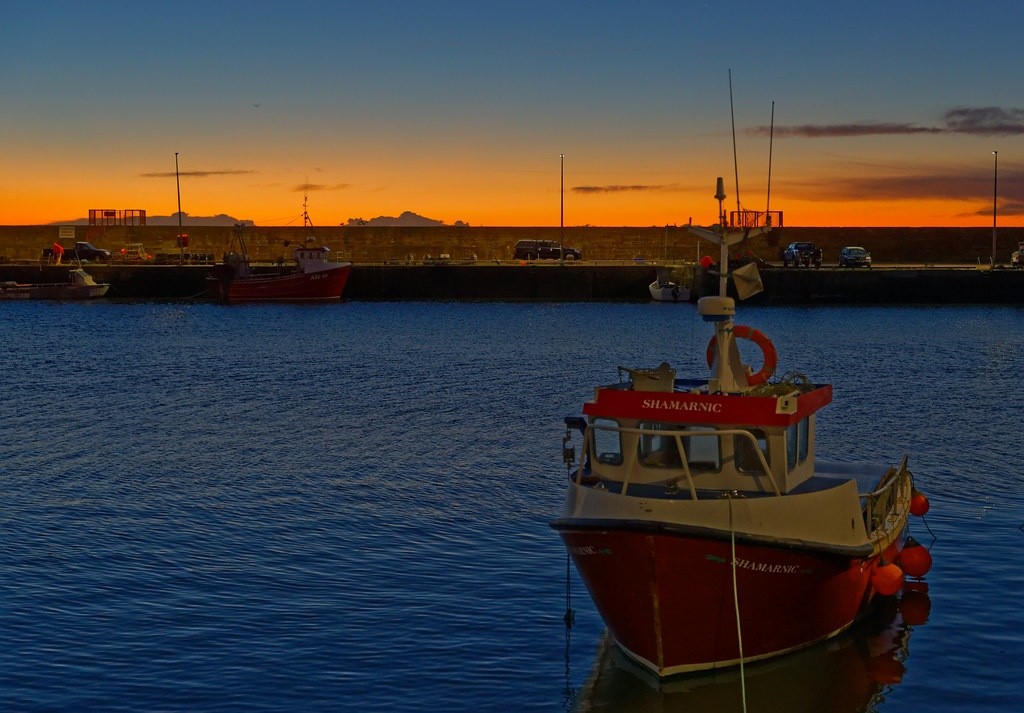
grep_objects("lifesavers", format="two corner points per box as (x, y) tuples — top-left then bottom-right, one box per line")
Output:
(705, 326), (777, 386)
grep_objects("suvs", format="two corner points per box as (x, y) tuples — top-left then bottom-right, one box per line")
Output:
(514, 240), (578, 261)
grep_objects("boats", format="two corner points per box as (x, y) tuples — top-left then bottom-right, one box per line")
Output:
(206, 197), (352, 305)
(0, 268), (110, 300)
(649, 279), (691, 302)
(546, 219), (930, 680)
(572, 585), (931, 712)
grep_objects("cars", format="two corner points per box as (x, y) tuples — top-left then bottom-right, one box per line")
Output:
(1011, 250), (1024, 267)
(839, 246), (872, 268)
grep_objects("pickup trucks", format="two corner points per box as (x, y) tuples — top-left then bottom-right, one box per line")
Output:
(784, 242), (823, 269)
(42, 241), (111, 261)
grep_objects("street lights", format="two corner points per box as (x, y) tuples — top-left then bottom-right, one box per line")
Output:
(991, 150), (998, 269)
(559, 153), (566, 266)
